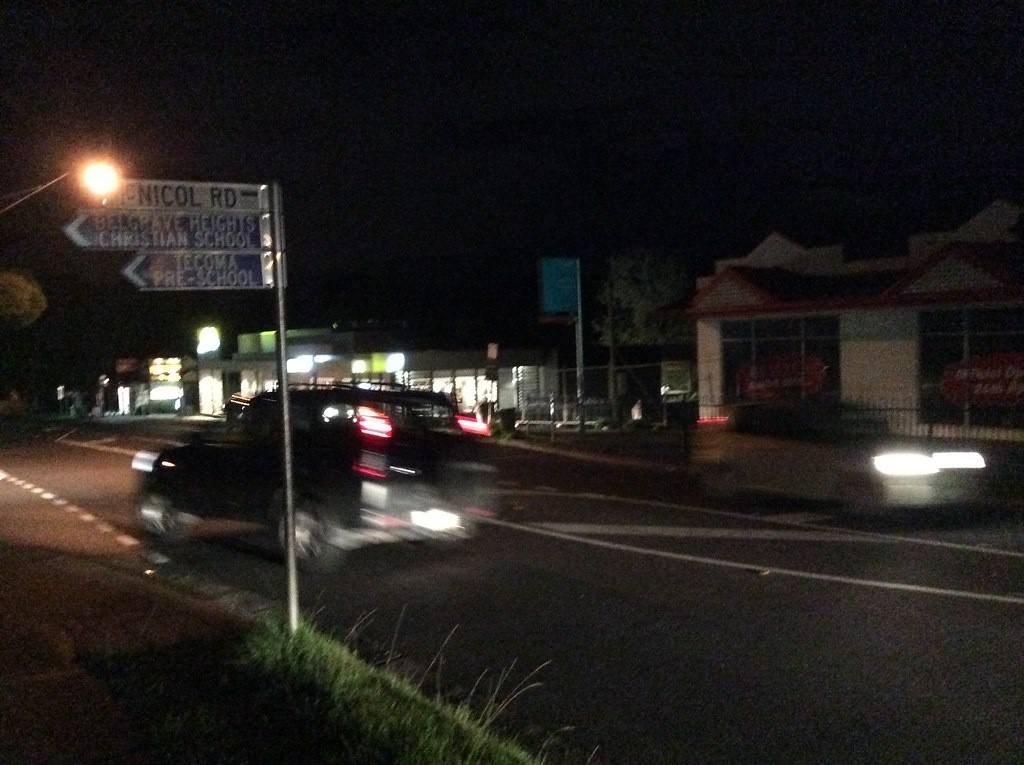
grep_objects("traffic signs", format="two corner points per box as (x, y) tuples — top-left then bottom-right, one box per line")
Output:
(65, 177), (275, 291)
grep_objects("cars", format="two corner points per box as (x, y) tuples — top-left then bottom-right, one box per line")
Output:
(694, 399), (992, 519)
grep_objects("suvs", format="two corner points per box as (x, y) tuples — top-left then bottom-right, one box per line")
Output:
(131, 379), (502, 572)
